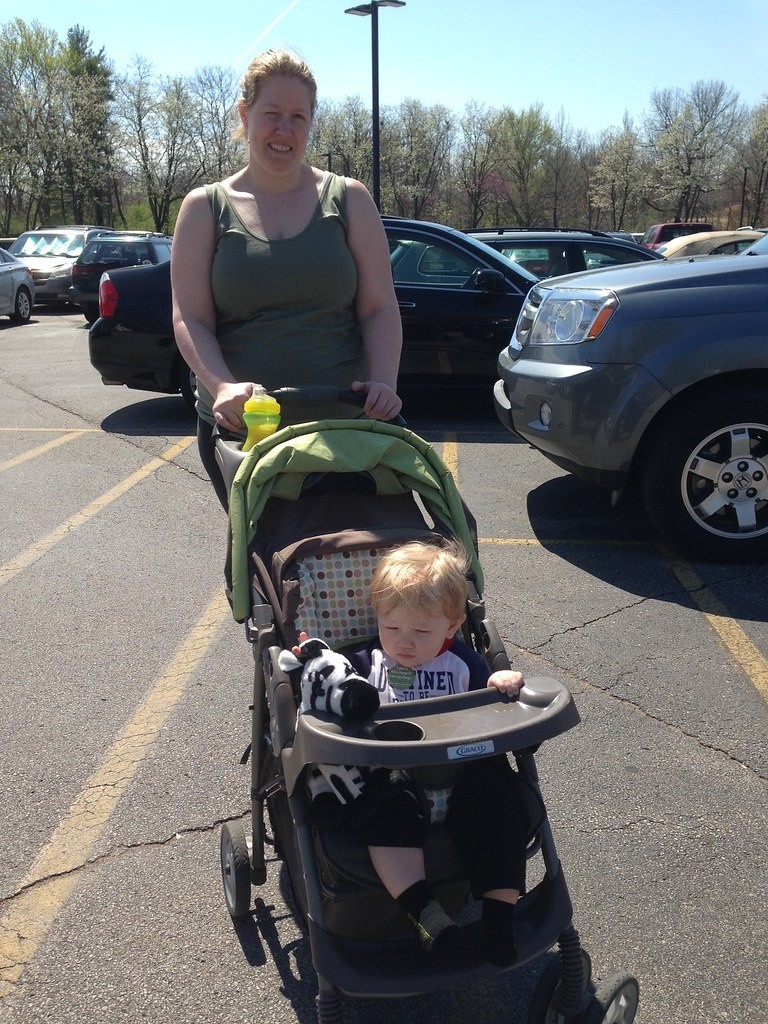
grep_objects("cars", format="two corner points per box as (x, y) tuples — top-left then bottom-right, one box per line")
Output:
(391, 221), (768, 285)
(88, 214), (541, 417)
(0, 246), (36, 326)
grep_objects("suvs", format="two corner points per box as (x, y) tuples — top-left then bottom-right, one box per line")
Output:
(493, 228), (768, 564)
(69, 229), (173, 325)
(6, 223), (116, 312)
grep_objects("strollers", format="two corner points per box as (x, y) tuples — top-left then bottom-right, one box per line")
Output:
(191, 379), (642, 1024)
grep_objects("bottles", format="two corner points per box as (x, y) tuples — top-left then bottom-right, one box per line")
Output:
(241, 386), (281, 452)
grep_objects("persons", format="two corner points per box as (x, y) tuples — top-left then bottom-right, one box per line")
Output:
(170, 47), (403, 518)
(291, 531), (523, 965)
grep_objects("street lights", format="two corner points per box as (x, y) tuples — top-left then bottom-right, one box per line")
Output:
(344, 0), (408, 213)
(739, 165), (752, 228)
(318, 149), (342, 172)
